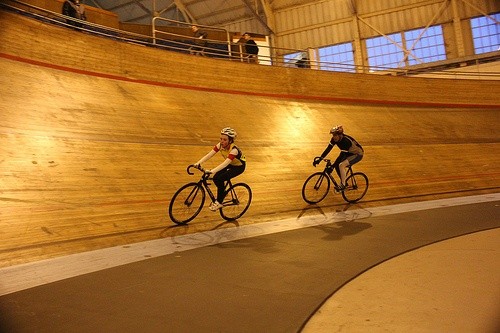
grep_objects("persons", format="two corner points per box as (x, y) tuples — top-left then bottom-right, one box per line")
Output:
(62, 0), (87, 31)
(244, 32), (259, 58)
(313, 125), (364, 191)
(188, 25), (208, 54)
(295, 52), (308, 68)
(194, 128), (246, 211)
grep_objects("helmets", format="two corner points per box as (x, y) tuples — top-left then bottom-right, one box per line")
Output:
(330, 125), (343, 134)
(221, 127), (238, 138)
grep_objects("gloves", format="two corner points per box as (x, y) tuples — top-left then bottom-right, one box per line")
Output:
(194, 162), (200, 168)
(313, 160), (319, 167)
(205, 172), (214, 178)
(326, 166), (333, 173)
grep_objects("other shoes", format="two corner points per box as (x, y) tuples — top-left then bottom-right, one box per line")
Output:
(334, 184), (346, 190)
(209, 200), (223, 210)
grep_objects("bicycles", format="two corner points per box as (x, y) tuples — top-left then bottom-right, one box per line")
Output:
(302, 156), (369, 204)
(169, 165), (252, 225)
